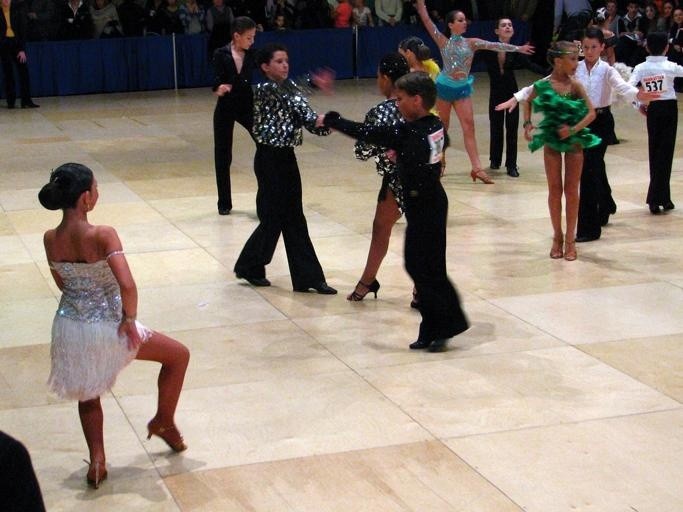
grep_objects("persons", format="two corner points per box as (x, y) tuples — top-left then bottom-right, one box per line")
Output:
(314, 37), (470, 350)
(206, 18), (337, 351)
(38, 161), (189, 489)
(413, 1), (683, 184)
(494, 29), (663, 261)
(0, 430), (45, 512)
(0, 1), (234, 110)
(253, 1), (402, 31)
(628, 32), (683, 214)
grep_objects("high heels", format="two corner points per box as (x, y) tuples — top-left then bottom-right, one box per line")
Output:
(86, 461), (108, 490)
(146, 416), (189, 452)
(346, 277), (381, 302)
(471, 168), (496, 184)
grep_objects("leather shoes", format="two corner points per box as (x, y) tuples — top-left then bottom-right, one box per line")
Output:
(575, 229), (600, 243)
(219, 207), (230, 215)
(293, 277), (338, 294)
(490, 161), (501, 170)
(408, 320), (454, 352)
(599, 197), (617, 225)
(236, 270), (272, 287)
(507, 166), (520, 178)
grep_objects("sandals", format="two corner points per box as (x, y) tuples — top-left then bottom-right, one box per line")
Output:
(549, 229), (564, 260)
(564, 233), (577, 262)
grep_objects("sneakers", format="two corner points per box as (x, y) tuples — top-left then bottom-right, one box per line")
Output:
(649, 200), (675, 214)
